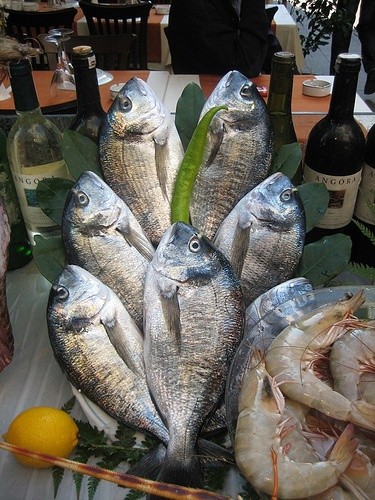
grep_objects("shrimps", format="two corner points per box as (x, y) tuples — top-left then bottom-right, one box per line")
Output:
(234, 286), (375, 500)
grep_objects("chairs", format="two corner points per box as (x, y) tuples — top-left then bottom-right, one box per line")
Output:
(1, 7), (78, 37)
(79, 1), (153, 70)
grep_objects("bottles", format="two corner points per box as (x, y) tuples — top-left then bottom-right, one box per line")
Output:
(354, 122), (375, 232)
(68, 45), (107, 148)
(7, 58), (71, 243)
(0, 130), (32, 272)
(265, 52), (297, 155)
(302, 54), (364, 247)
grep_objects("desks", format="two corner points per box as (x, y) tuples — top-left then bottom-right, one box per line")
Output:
(0, 0), (375, 500)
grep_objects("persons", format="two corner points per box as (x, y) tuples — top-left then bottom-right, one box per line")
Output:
(357, 0), (375, 94)
(169, 0), (282, 79)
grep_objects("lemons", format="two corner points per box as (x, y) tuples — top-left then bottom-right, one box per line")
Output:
(2, 407), (79, 468)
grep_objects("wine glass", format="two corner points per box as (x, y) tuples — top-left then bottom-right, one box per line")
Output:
(47, 27), (76, 80)
(46, 35), (75, 101)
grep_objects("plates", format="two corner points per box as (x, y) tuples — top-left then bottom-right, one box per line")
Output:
(58, 68), (114, 90)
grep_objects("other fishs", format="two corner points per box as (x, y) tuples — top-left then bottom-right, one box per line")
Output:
(46, 170), (238, 485)
(142, 220), (245, 490)
(97, 69), (316, 452)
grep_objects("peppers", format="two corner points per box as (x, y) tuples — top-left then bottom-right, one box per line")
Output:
(170, 105), (230, 225)
